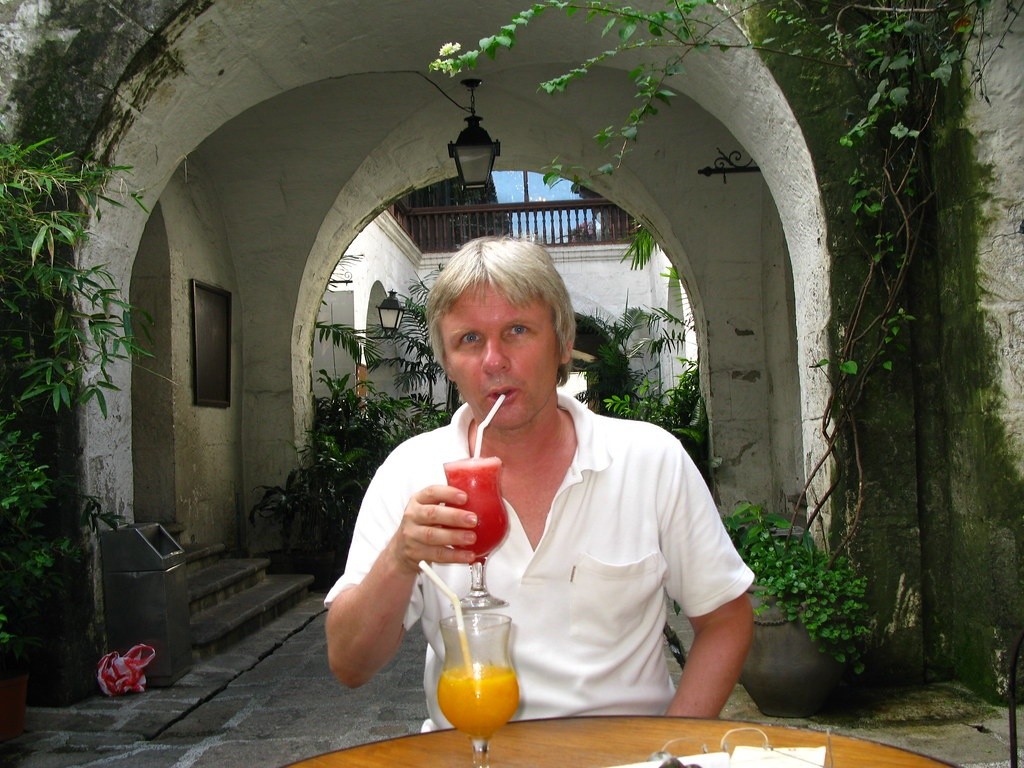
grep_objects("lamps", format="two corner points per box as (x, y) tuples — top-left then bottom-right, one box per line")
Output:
(360, 289), (407, 340)
(447, 79), (501, 193)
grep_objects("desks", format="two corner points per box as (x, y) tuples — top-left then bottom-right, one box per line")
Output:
(278, 714), (966, 768)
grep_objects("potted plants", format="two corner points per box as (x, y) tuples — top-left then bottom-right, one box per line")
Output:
(730, 502), (872, 719)
(247, 431), (362, 592)
(0, 410), (65, 741)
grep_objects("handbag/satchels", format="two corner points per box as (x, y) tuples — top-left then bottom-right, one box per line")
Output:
(96, 645), (157, 696)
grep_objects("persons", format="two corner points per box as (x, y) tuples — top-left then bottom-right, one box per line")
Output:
(323, 235), (757, 720)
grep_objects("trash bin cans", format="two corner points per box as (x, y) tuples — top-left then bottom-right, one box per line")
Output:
(99, 523), (193, 688)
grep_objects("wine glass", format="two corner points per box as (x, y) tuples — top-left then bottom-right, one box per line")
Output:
(443, 456), (509, 611)
(438, 613), (518, 768)
